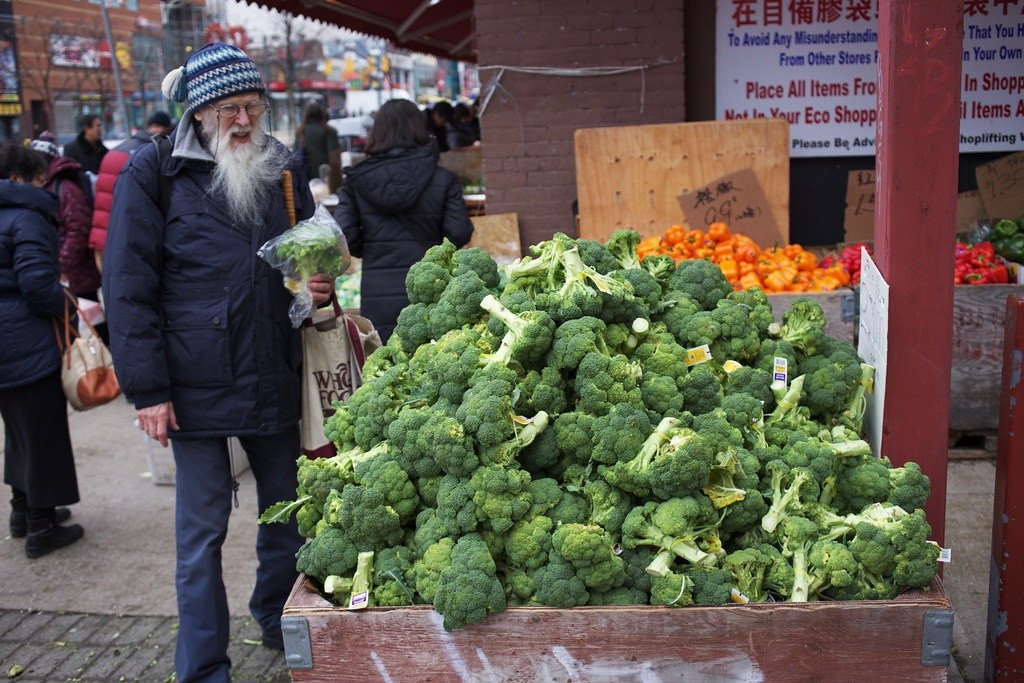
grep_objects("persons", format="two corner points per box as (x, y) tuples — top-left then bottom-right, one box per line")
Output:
(422, 101), (481, 151)
(292, 101), (340, 182)
(88, 112), (171, 252)
(333, 99), (475, 346)
(26, 129), (93, 283)
(62, 114), (109, 175)
(0, 144), (78, 559)
(101, 42), (333, 683)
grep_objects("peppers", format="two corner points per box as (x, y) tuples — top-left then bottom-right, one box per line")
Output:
(639, 219), (1024, 294)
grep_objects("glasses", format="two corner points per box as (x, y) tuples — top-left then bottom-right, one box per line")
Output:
(35, 176), (47, 186)
(208, 95), (270, 118)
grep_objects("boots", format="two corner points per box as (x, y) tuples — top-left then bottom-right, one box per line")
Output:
(25, 509), (84, 558)
(9, 499), (71, 538)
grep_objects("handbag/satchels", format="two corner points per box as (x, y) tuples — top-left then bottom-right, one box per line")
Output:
(55, 289), (122, 412)
(299, 289), (383, 461)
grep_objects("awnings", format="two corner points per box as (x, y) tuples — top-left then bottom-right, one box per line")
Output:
(236, 0), (477, 65)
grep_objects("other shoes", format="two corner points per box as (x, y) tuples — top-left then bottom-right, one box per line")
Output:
(262, 632), (284, 651)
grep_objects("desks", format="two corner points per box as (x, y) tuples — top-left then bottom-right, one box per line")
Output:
(281, 573), (955, 683)
(767, 254), (1024, 452)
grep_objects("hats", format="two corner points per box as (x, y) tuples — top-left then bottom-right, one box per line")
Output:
(160, 43), (265, 114)
(28, 131), (59, 159)
(148, 112), (171, 128)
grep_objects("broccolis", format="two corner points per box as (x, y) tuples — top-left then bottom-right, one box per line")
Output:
(258, 230), (940, 629)
